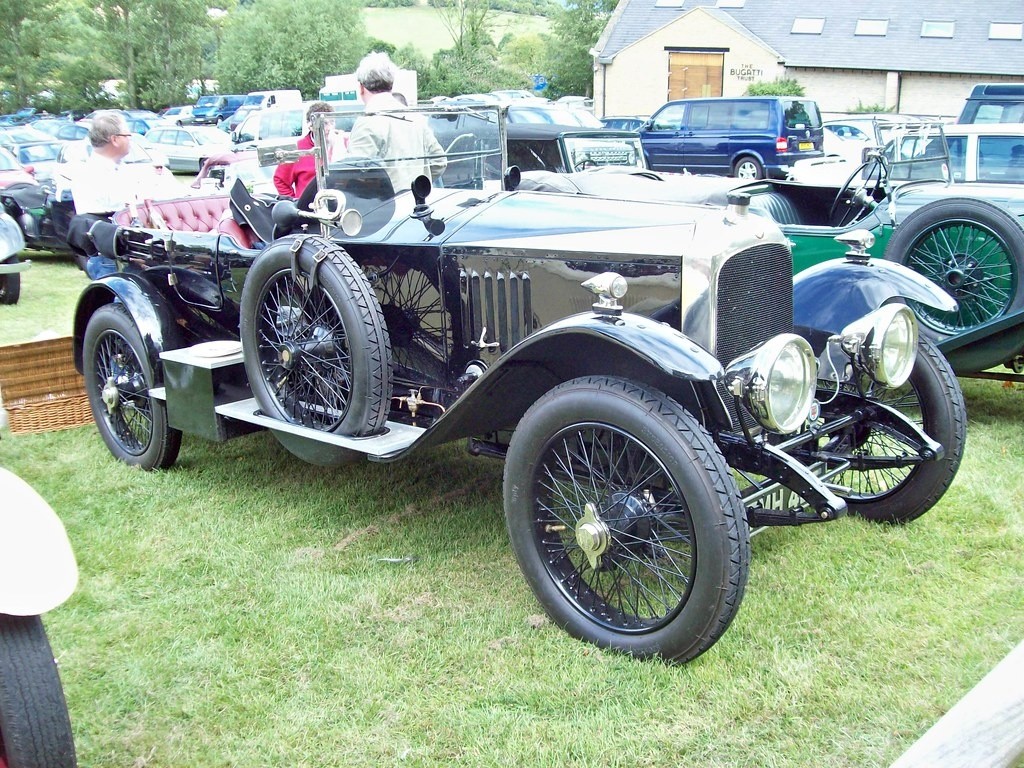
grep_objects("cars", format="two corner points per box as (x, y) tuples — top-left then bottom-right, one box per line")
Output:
(0, 66), (1024, 662)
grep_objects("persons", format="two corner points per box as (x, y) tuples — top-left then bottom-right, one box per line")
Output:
(69, 107), (156, 387)
(273, 50), (448, 211)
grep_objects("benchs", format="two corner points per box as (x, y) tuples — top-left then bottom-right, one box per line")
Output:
(113, 193), (261, 251)
(749, 191), (830, 226)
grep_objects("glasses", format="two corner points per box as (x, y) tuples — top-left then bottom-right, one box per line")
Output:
(153, 165), (163, 169)
